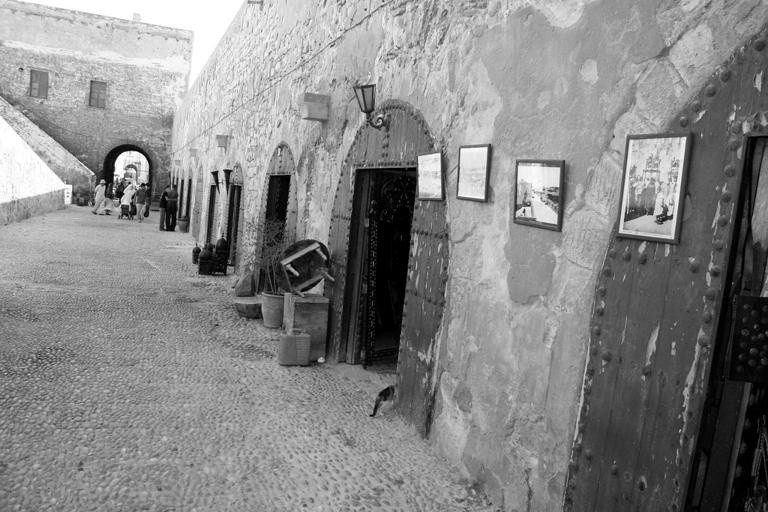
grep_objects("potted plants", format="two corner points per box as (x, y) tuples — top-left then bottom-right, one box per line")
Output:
(246, 212), (288, 329)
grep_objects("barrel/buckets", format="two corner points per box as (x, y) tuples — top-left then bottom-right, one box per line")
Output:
(277, 326), (311, 366)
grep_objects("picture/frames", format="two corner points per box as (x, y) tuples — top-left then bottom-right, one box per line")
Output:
(514, 159), (565, 232)
(456, 144), (491, 202)
(417, 150), (444, 201)
(615, 135), (691, 243)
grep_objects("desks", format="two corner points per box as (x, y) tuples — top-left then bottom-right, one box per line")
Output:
(276, 240), (336, 297)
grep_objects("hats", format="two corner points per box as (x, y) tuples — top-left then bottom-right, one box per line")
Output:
(100, 180), (106, 183)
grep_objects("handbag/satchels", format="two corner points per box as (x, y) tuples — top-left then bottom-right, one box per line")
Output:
(144, 207), (149, 217)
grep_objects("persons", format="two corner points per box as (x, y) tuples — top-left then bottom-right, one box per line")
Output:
(91, 178), (152, 222)
(165, 185), (178, 231)
(158, 185), (177, 231)
(625, 174), (676, 226)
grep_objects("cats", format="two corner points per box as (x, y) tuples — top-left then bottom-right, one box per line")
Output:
(369, 385), (396, 418)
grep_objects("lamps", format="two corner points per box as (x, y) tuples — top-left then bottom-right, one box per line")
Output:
(223, 168), (234, 197)
(296, 93), (331, 122)
(190, 148), (198, 156)
(355, 81), (385, 129)
(215, 135), (228, 147)
(210, 169), (224, 193)
